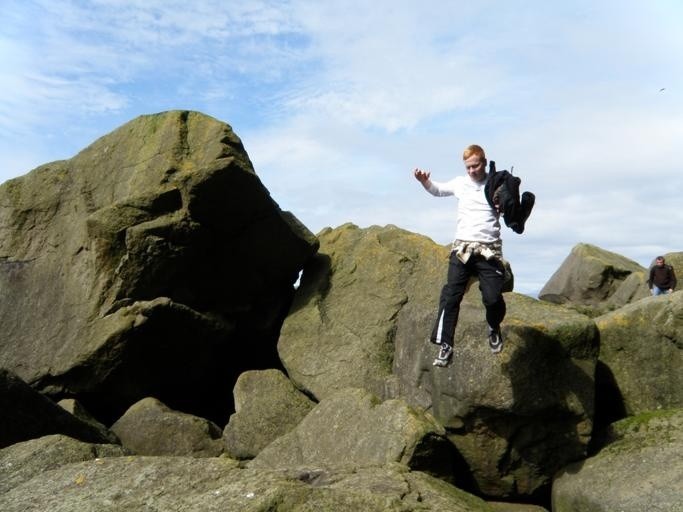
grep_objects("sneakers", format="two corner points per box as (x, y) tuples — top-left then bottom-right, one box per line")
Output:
(432, 341), (453, 367)
(488, 326), (503, 354)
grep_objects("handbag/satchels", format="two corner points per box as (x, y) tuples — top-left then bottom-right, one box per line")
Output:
(500, 260), (514, 293)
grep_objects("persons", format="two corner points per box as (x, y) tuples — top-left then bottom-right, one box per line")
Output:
(414, 144), (506, 367)
(647, 256), (677, 295)
